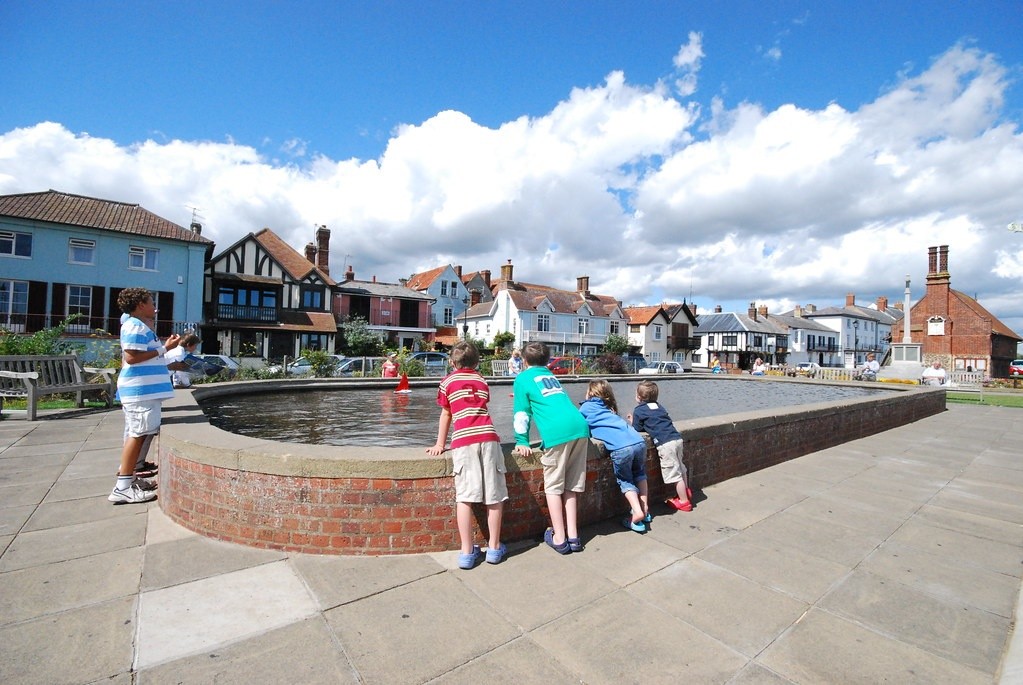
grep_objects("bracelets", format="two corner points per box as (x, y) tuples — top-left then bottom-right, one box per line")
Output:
(436, 442), (445, 449)
(155, 345), (168, 357)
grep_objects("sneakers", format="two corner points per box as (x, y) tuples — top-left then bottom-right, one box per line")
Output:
(485, 542), (507, 564)
(132, 476), (157, 491)
(108, 487), (157, 504)
(458, 544), (481, 569)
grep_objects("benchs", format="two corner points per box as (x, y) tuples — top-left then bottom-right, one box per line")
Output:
(765, 366), (797, 377)
(810, 367), (862, 379)
(917, 370), (984, 401)
(0, 350), (118, 421)
(491, 359), (510, 376)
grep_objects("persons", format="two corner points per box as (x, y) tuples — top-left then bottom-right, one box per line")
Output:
(922, 360), (946, 386)
(114, 310), (201, 477)
(107, 286), (182, 503)
(382, 351), (401, 378)
(425, 340), (509, 569)
(861, 352), (880, 373)
(512, 342), (590, 556)
(580, 379), (648, 524)
(753, 358), (766, 373)
(627, 381), (696, 512)
(711, 352), (722, 374)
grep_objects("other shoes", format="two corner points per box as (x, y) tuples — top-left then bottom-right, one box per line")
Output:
(117, 461), (158, 476)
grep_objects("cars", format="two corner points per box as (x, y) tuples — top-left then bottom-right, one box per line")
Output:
(265, 354), (347, 378)
(795, 361), (821, 378)
(193, 354), (243, 369)
(337, 356), (399, 377)
(638, 360), (685, 374)
(1008, 359), (1023, 376)
(623, 356), (648, 373)
(405, 352), (450, 376)
(547, 356), (583, 375)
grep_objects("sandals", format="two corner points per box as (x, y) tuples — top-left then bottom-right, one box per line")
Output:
(685, 487), (694, 501)
(666, 498), (692, 510)
(544, 526), (582, 555)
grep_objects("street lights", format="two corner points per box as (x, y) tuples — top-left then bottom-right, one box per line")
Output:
(461, 294), (471, 340)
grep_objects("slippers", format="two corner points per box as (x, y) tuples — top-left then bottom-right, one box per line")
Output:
(622, 518), (646, 533)
(629, 508), (652, 522)
